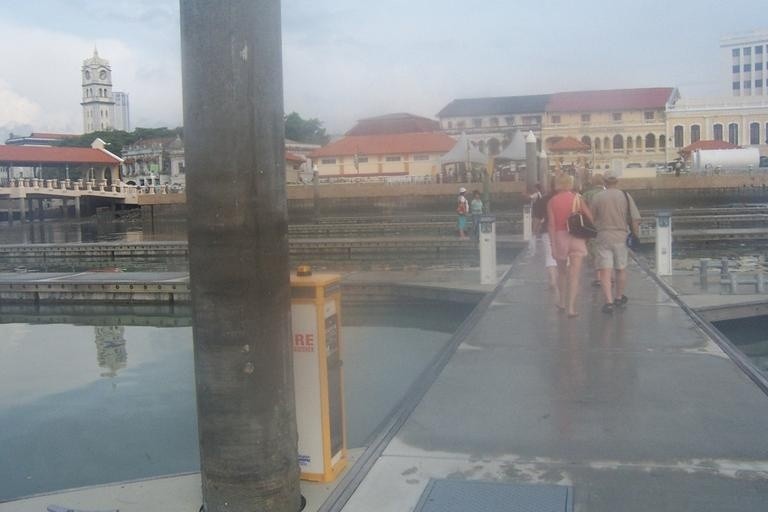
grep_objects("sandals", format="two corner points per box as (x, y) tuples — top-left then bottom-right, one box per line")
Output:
(601, 303), (612, 312)
(615, 295), (627, 306)
(591, 278), (615, 287)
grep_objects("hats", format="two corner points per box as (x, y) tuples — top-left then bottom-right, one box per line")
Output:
(458, 188), (467, 193)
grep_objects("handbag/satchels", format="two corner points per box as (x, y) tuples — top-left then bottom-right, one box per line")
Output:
(456, 204), (466, 214)
(533, 199), (544, 215)
(626, 233), (639, 250)
(566, 214), (597, 238)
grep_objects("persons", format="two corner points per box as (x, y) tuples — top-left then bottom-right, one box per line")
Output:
(296, 160), (306, 184)
(19, 172), (23, 178)
(705, 161), (713, 178)
(437, 156), (642, 319)
(165, 182), (169, 194)
(675, 160), (681, 177)
(150, 170), (155, 176)
